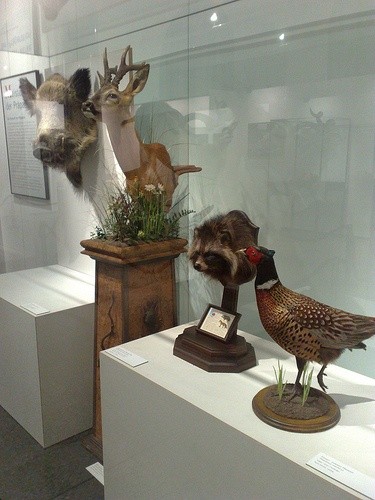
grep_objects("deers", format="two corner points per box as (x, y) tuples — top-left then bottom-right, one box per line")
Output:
(82, 45), (202, 212)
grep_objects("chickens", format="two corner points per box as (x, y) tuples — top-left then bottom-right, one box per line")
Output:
(234, 246), (375, 402)
